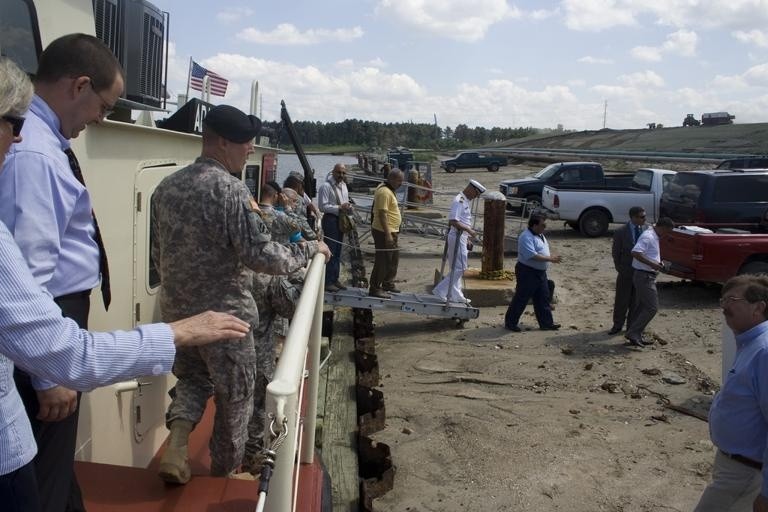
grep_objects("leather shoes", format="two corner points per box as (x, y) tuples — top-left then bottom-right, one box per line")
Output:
(608, 326), (654, 347)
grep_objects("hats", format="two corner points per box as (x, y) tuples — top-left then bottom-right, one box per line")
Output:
(470, 178), (485, 193)
(266, 179), (281, 192)
(289, 170), (304, 182)
(205, 104), (262, 144)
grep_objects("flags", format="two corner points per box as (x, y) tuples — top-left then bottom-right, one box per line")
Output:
(188, 61), (229, 97)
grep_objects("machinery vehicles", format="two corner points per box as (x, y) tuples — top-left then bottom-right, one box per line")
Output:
(701, 113), (735, 125)
(682, 113), (700, 127)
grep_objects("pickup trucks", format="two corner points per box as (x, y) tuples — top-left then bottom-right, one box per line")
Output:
(541, 168), (680, 237)
(439, 153), (510, 173)
(653, 224), (768, 287)
(498, 159), (635, 218)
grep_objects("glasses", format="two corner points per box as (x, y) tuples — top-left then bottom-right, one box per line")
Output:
(720, 296), (747, 305)
(2, 113), (26, 136)
(640, 214), (647, 219)
(90, 85), (115, 118)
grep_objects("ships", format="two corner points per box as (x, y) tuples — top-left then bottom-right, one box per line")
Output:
(0, 0), (480, 511)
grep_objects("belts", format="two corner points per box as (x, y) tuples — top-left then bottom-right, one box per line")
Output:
(643, 269), (656, 274)
(719, 448), (762, 469)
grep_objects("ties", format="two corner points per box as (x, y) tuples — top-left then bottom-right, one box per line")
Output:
(634, 225), (641, 244)
(64, 147), (111, 311)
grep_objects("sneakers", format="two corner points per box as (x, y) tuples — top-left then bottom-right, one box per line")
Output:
(369, 288), (400, 298)
(326, 281), (347, 291)
(230, 471), (256, 481)
(505, 325), (521, 331)
(158, 442), (190, 484)
(433, 288), (448, 303)
(454, 296), (470, 304)
(541, 324), (562, 330)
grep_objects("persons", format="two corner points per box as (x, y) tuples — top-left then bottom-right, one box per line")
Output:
(433, 179), (487, 305)
(369, 169), (405, 299)
(690, 274), (768, 512)
(0, 33), (126, 510)
(606, 206), (647, 335)
(150, 104), (332, 484)
(0, 57), (250, 509)
(624, 217), (674, 348)
(504, 212), (562, 332)
(317, 163), (350, 293)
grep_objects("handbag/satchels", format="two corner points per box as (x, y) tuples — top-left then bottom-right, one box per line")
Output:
(339, 209), (360, 232)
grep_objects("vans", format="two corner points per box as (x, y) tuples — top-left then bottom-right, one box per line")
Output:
(659, 169), (767, 230)
(714, 155), (766, 168)
